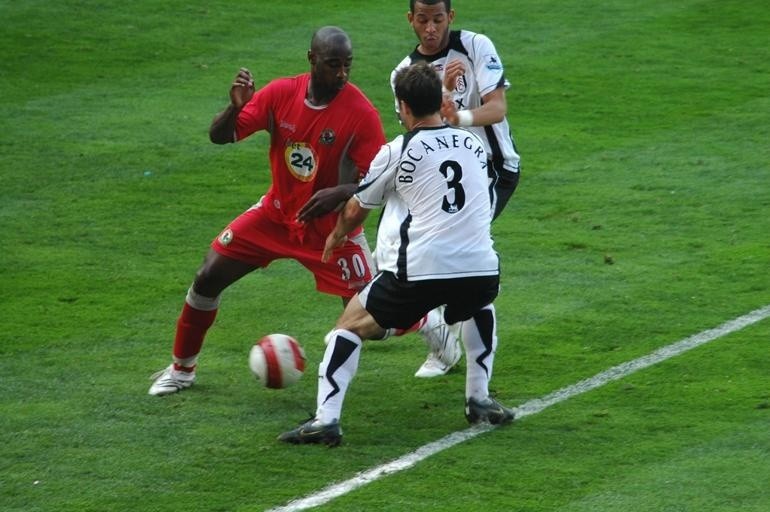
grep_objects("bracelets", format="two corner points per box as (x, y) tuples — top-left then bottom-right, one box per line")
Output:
(457, 110), (474, 128)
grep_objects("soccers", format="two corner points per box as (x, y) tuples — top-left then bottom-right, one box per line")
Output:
(248, 334), (307, 389)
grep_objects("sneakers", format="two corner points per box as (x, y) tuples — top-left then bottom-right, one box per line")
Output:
(148, 363), (195, 396)
(276, 412), (342, 448)
(414, 306), (463, 379)
(464, 393), (514, 425)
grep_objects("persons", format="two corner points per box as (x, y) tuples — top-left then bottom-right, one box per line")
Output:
(146, 25), (458, 398)
(372, 0), (521, 378)
(274, 60), (515, 448)
